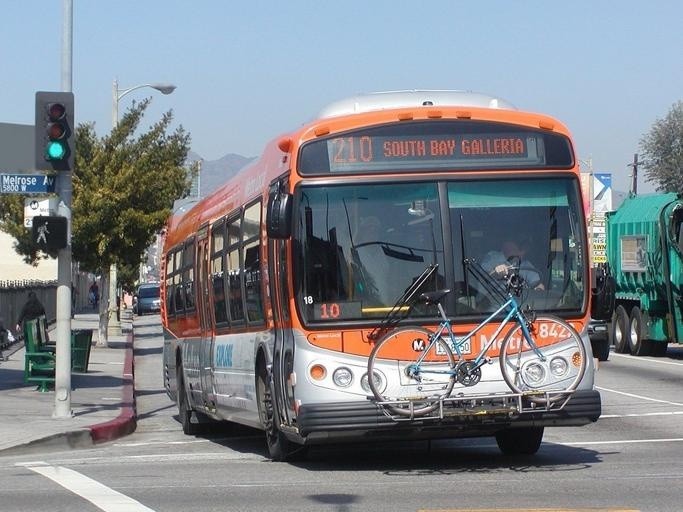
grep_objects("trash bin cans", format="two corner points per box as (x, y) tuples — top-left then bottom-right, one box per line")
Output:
(70, 329), (93, 373)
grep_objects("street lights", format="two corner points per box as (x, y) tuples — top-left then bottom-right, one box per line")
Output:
(107, 78), (176, 338)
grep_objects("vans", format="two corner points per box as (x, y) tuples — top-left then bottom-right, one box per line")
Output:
(136, 284), (161, 315)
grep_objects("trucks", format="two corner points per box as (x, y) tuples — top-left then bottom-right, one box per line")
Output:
(604, 190), (681, 366)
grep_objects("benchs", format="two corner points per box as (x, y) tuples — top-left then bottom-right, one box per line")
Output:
(23, 315), (56, 393)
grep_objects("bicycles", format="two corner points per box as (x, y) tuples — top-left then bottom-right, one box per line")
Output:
(364, 255), (590, 417)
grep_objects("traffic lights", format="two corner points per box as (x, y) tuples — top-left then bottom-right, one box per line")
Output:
(32, 216), (66, 250)
(35, 92), (75, 170)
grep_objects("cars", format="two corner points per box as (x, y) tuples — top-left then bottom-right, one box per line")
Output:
(585, 315), (609, 362)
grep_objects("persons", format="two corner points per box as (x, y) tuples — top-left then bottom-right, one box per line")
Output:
(88, 281), (99, 309)
(70, 282), (80, 320)
(473, 229), (545, 305)
(15, 291), (44, 352)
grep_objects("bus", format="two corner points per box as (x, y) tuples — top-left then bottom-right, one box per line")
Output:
(157, 88), (616, 472)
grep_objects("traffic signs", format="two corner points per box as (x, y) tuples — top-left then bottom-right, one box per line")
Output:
(0, 174), (55, 194)
